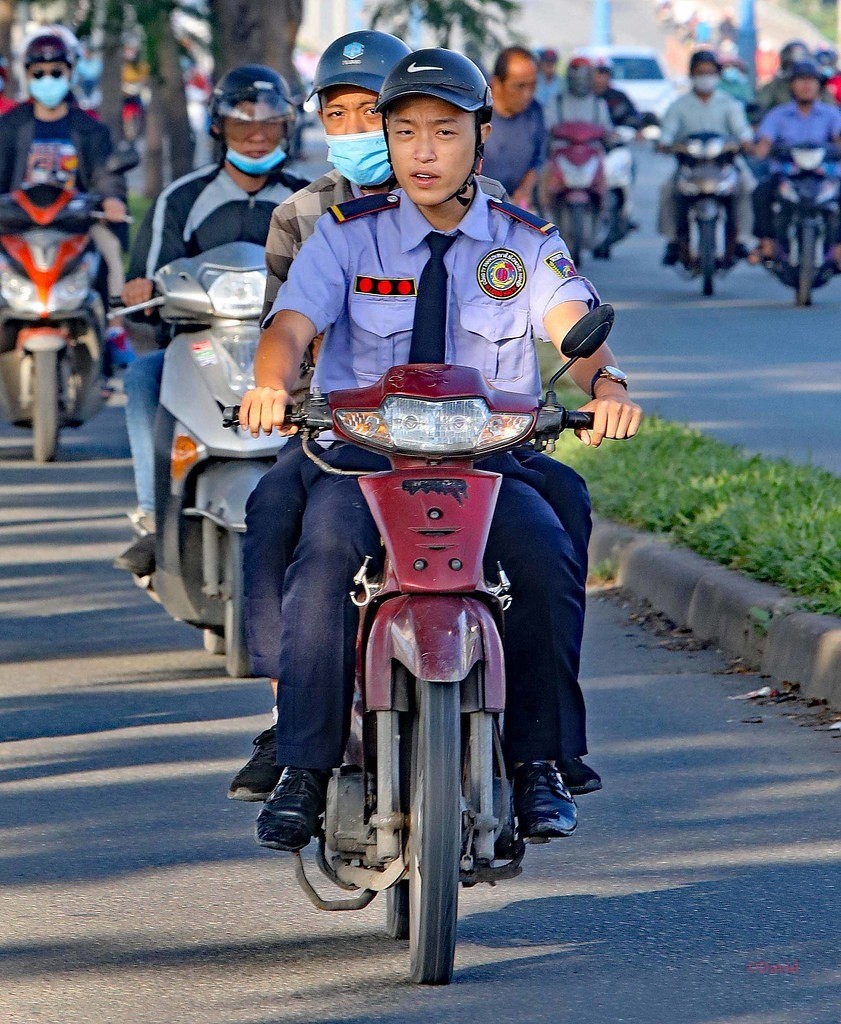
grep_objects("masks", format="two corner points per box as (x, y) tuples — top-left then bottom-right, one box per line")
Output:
(324, 130), (393, 186)
(569, 76), (593, 97)
(694, 76), (720, 92)
(27, 75), (69, 110)
(226, 145), (286, 174)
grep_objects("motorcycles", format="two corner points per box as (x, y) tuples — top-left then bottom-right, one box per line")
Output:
(220, 298), (627, 986)
(651, 129), (763, 299)
(747, 140), (840, 308)
(2, 140), (138, 459)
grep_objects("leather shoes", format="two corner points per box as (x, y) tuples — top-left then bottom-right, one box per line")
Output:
(112, 533), (157, 573)
(255, 766), (334, 851)
(513, 761), (577, 837)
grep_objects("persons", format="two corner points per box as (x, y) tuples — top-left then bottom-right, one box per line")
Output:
(658, 43), (840, 275)
(482, 45), (546, 218)
(114, 66), (313, 573)
(0, 24), (137, 343)
(219, 36), (642, 854)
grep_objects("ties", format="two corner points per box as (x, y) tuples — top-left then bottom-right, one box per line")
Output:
(409, 233), (461, 364)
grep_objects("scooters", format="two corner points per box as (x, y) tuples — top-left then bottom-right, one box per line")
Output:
(104, 241), (289, 682)
(548, 121), (641, 270)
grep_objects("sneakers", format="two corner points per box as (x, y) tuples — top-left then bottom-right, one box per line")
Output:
(228, 725), (284, 800)
(556, 758), (603, 795)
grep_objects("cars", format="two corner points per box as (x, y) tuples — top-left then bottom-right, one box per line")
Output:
(607, 55), (676, 120)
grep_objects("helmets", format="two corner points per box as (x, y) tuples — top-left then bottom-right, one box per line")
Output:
(209, 64), (297, 122)
(791, 61), (823, 80)
(780, 41), (808, 62)
(23, 35), (73, 69)
(689, 51), (721, 74)
(569, 56), (591, 70)
(306, 30), (413, 102)
(374, 48), (493, 125)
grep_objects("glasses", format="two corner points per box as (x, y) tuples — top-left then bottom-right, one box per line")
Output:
(32, 69), (63, 78)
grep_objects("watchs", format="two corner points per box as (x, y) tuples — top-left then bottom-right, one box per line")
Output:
(587, 365), (629, 401)
